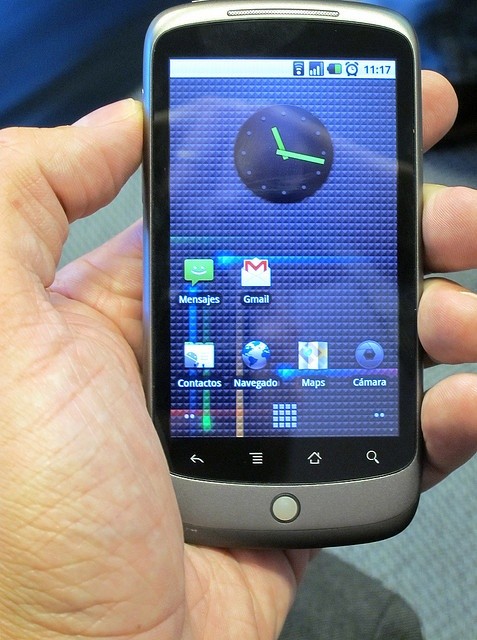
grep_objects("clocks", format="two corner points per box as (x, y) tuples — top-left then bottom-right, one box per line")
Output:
(233, 105), (333, 204)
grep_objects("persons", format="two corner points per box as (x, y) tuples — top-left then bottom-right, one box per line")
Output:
(0, 68), (477, 640)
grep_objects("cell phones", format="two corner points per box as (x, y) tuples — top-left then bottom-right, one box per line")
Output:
(142, 0), (423, 550)
